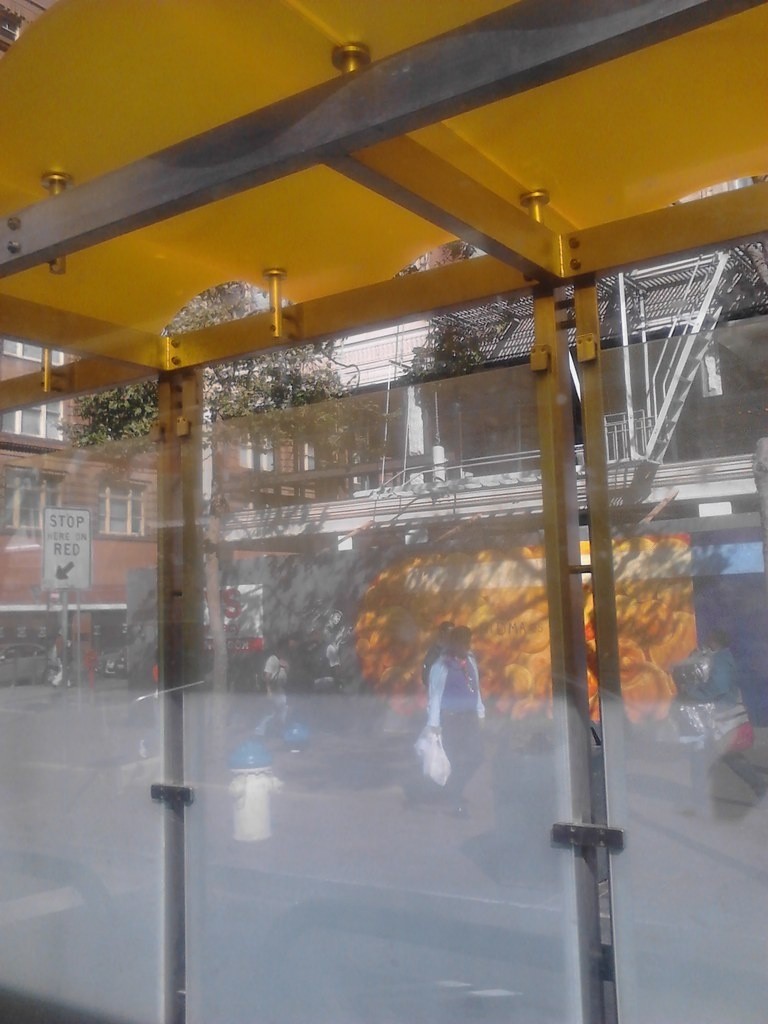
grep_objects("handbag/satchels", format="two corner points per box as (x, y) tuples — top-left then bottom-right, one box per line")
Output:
(414, 729), (451, 786)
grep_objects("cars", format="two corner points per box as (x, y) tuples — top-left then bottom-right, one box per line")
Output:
(94, 641), (126, 679)
(1, 641), (50, 686)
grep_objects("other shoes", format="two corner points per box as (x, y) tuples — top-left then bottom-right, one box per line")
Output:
(255, 727), (266, 736)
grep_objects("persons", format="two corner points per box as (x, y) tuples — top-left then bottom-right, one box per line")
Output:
(254, 637), (300, 746)
(697, 631), (767, 809)
(421, 620), (486, 809)
